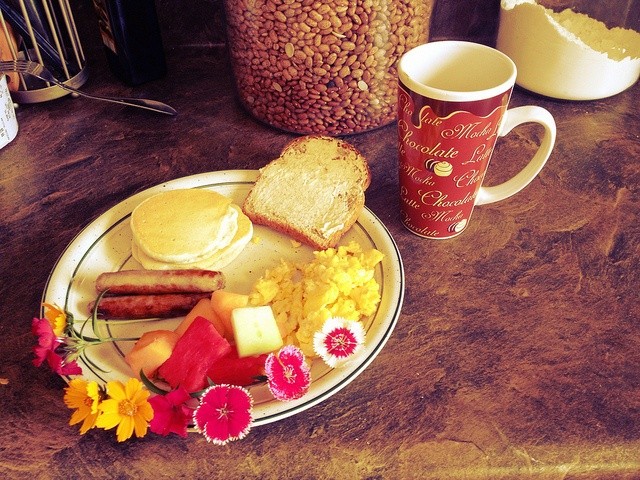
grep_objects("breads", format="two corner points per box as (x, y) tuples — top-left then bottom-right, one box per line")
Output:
(243, 135), (371, 249)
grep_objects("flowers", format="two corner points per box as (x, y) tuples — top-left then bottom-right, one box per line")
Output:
(29, 314), (142, 375)
(41, 303), (162, 334)
(59, 380), (106, 433)
(264, 347), (311, 401)
(313, 318), (366, 372)
(192, 384), (254, 446)
(148, 385), (195, 438)
(95, 380), (154, 443)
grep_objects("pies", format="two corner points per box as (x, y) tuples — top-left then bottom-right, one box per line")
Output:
(132, 188), (235, 264)
(132, 205), (254, 270)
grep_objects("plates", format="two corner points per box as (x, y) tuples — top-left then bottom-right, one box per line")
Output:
(39, 170), (406, 433)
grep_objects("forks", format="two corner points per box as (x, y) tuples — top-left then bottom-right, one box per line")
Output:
(1, 60), (177, 116)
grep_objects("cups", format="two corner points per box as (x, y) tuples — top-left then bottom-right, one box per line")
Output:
(397, 41), (556, 240)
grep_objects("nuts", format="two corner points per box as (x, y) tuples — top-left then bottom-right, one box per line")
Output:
(224, 1), (433, 138)
